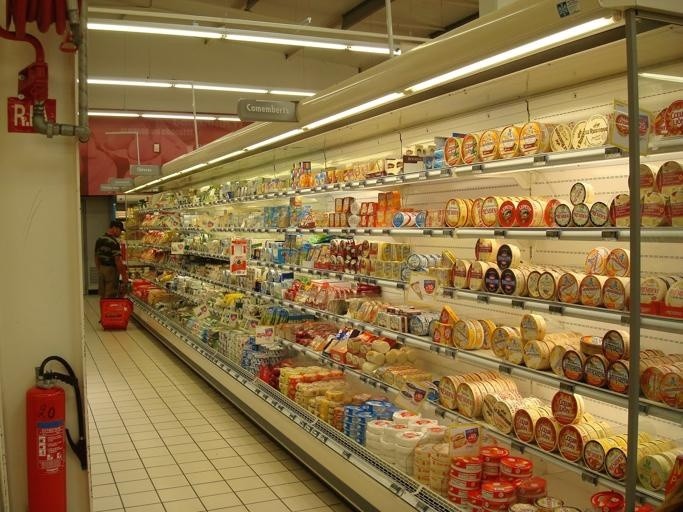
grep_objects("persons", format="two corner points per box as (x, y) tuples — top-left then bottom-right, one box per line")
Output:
(95, 220), (128, 298)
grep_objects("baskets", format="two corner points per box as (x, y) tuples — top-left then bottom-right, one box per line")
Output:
(99, 297), (135, 331)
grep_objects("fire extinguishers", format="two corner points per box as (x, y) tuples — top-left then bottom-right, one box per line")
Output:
(26, 355), (88, 512)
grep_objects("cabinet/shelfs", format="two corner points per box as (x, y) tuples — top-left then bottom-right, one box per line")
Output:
(130, 1), (683, 511)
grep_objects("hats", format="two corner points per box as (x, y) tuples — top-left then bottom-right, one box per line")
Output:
(110, 220), (126, 232)
